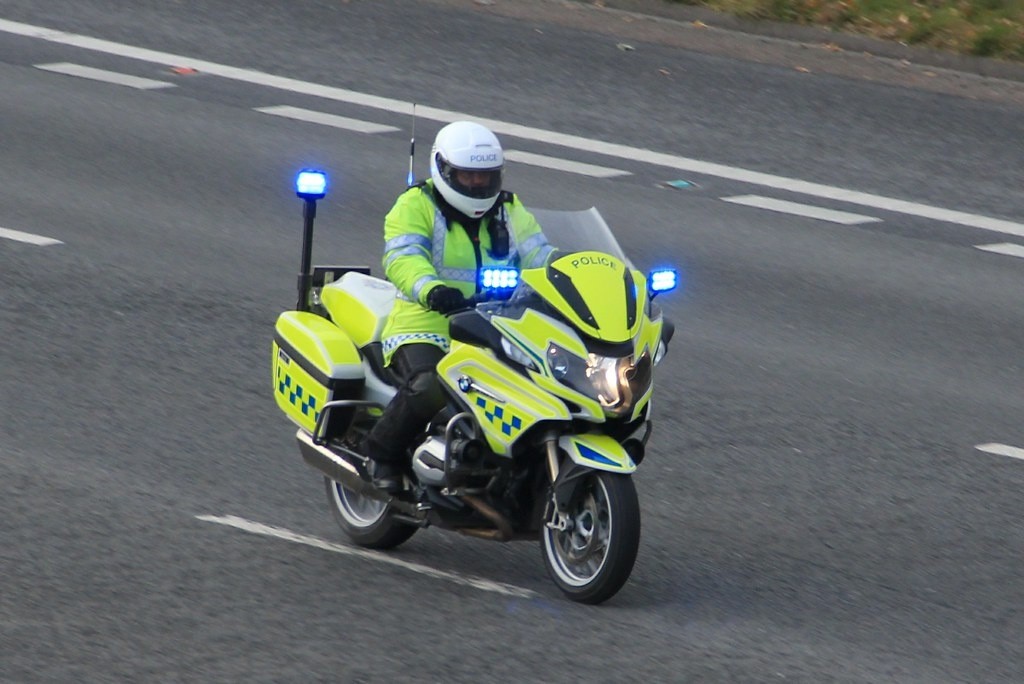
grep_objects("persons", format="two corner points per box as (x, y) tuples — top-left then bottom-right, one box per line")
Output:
(348, 119), (577, 494)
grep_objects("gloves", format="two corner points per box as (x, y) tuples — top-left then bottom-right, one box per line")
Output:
(426, 285), (467, 315)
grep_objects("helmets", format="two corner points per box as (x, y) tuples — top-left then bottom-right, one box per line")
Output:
(431, 121), (504, 218)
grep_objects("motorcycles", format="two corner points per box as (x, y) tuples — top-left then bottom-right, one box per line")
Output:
(271, 168), (680, 604)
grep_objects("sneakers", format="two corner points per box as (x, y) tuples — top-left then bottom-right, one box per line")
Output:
(364, 458), (403, 489)
